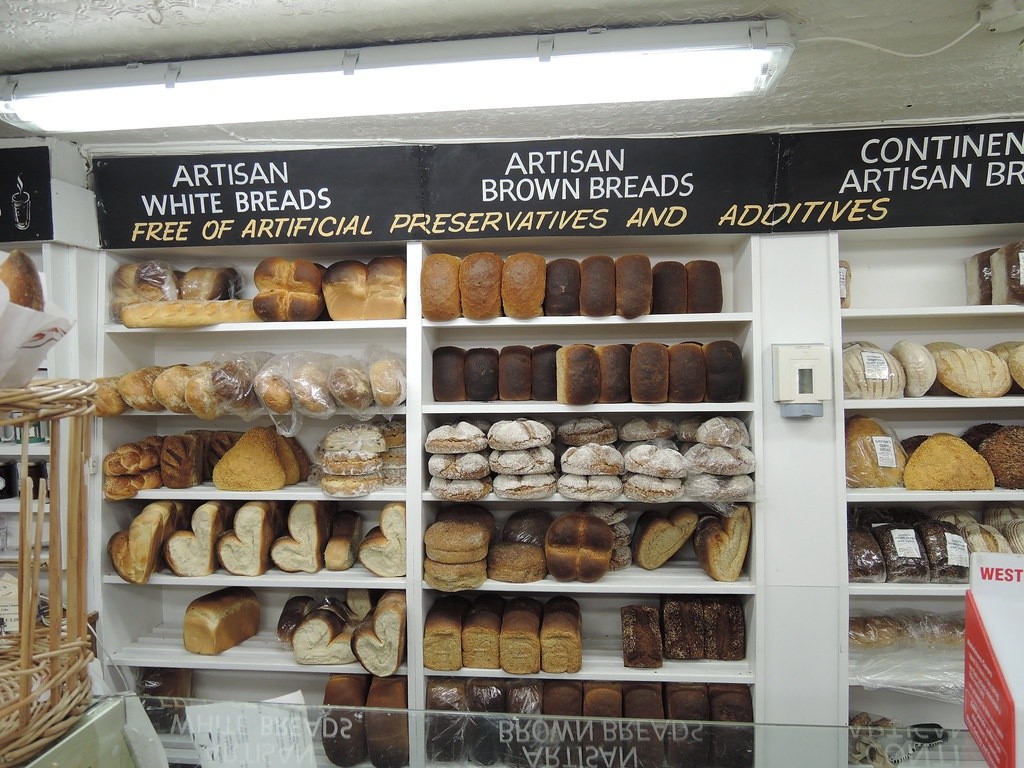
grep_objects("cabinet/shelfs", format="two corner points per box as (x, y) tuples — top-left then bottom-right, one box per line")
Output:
(0, 243), (69, 570)
(90, 235), (765, 768)
(828, 223), (1024, 767)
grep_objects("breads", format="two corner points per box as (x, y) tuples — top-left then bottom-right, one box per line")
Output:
(0, 249), (45, 314)
(421, 253), (756, 768)
(840, 242), (1024, 651)
(92, 255), (407, 768)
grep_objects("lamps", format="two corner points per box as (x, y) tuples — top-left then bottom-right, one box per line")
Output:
(0, 18), (793, 141)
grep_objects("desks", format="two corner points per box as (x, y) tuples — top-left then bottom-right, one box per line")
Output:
(22, 690), (992, 768)
